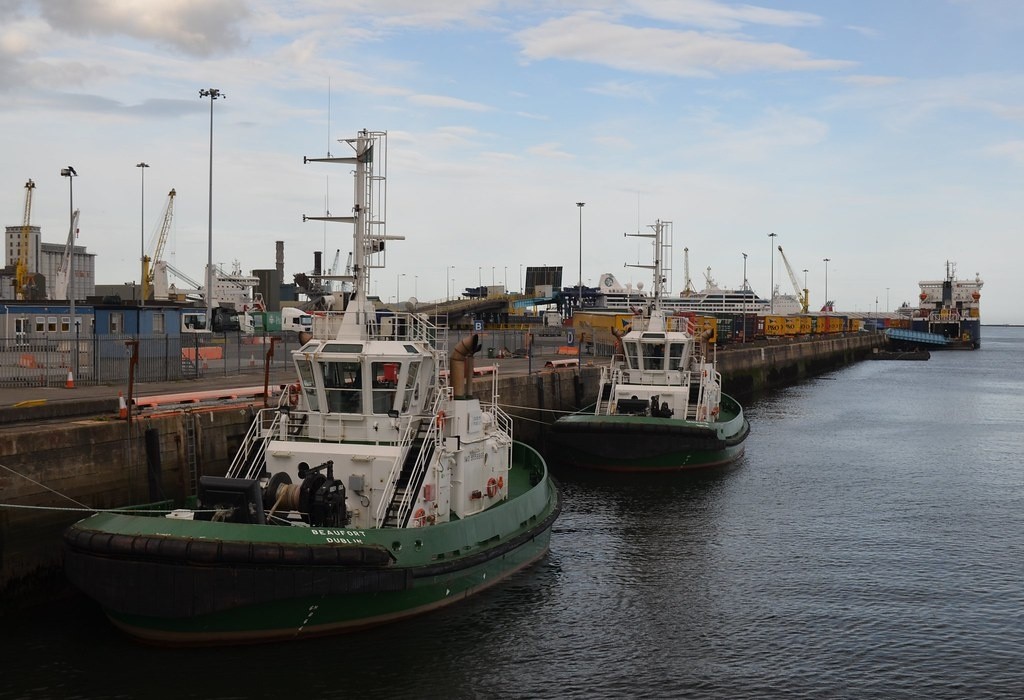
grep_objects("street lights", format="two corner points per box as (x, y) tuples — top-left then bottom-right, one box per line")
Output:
(742, 253), (748, 344)
(768, 233), (779, 315)
(803, 269), (809, 315)
(823, 258), (830, 313)
(373, 264), (547, 311)
(576, 202), (586, 308)
(60, 165), (82, 388)
(875, 297), (878, 335)
(136, 163), (150, 306)
(886, 287), (890, 312)
(199, 88), (227, 331)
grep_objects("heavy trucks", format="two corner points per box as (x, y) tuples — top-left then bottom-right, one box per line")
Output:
(162, 306), (240, 333)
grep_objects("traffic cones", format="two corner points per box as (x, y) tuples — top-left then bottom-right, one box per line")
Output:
(65, 367), (77, 389)
(201, 353), (209, 369)
(249, 352), (255, 367)
(114, 389), (132, 420)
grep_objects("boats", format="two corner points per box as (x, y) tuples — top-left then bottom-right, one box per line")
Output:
(180, 312), (223, 360)
(551, 311), (751, 482)
(61, 311), (565, 665)
(911, 259), (984, 350)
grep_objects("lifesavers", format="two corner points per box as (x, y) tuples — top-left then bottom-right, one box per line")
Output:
(436, 409), (449, 431)
(486, 477), (499, 499)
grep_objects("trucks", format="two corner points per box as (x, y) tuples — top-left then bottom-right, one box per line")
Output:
(250, 306), (313, 343)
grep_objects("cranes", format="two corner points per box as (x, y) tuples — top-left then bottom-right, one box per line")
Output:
(778, 246), (810, 314)
